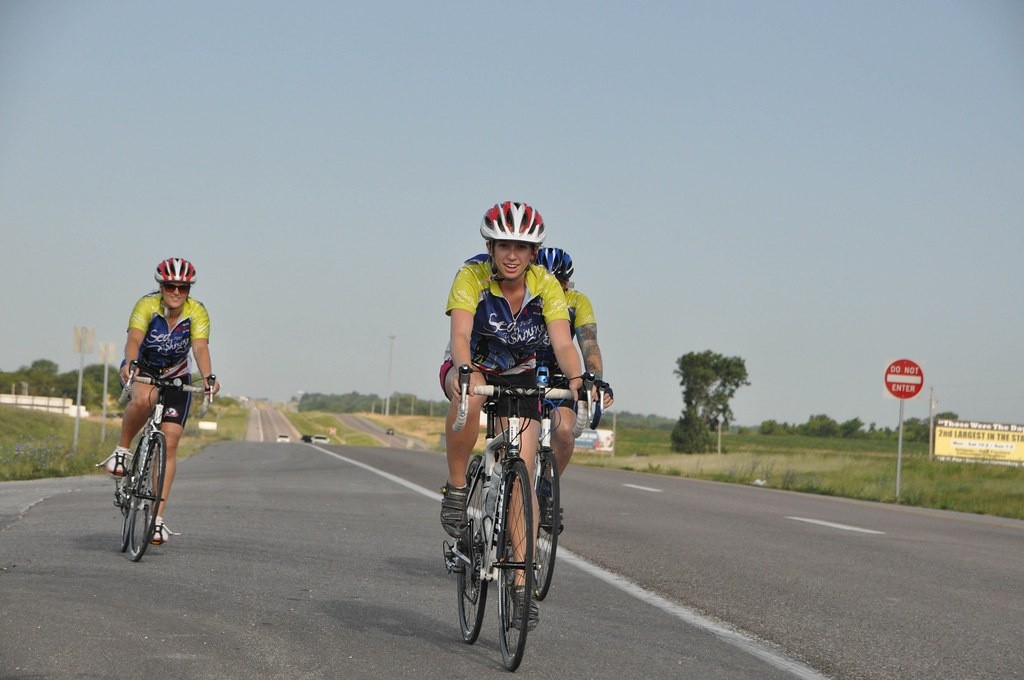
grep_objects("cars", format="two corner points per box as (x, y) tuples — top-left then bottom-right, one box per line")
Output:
(274, 434), (291, 443)
(310, 434), (331, 445)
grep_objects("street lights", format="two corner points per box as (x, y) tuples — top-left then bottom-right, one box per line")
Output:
(385, 333), (396, 416)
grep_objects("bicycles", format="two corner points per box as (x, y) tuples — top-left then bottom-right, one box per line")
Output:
(93, 359), (217, 562)
(529, 370), (611, 604)
(441, 361), (594, 674)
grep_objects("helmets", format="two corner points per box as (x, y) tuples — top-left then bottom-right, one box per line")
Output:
(155, 257), (197, 285)
(535, 247), (574, 283)
(480, 200), (545, 244)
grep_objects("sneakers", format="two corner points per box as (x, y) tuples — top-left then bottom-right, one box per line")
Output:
(94, 444), (134, 475)
(508, 580), (539, 630)
(439, 479), (470, 538)
(147, 516), (181, 544)
(535, 476), (564, 535)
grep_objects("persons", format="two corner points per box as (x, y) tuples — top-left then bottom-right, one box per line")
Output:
(439, 201), (604, 630)
(104, 257), (220, 545)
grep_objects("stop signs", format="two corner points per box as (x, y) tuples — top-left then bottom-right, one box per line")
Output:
(884, 358), (925, 401)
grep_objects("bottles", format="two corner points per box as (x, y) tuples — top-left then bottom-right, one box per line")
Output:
(485, 463), (502, 517)
(482, 476), (490, 521)
(137, 436), (151, 475)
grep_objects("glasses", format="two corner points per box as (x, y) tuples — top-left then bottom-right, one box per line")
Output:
(160, 283), (189, 295)
